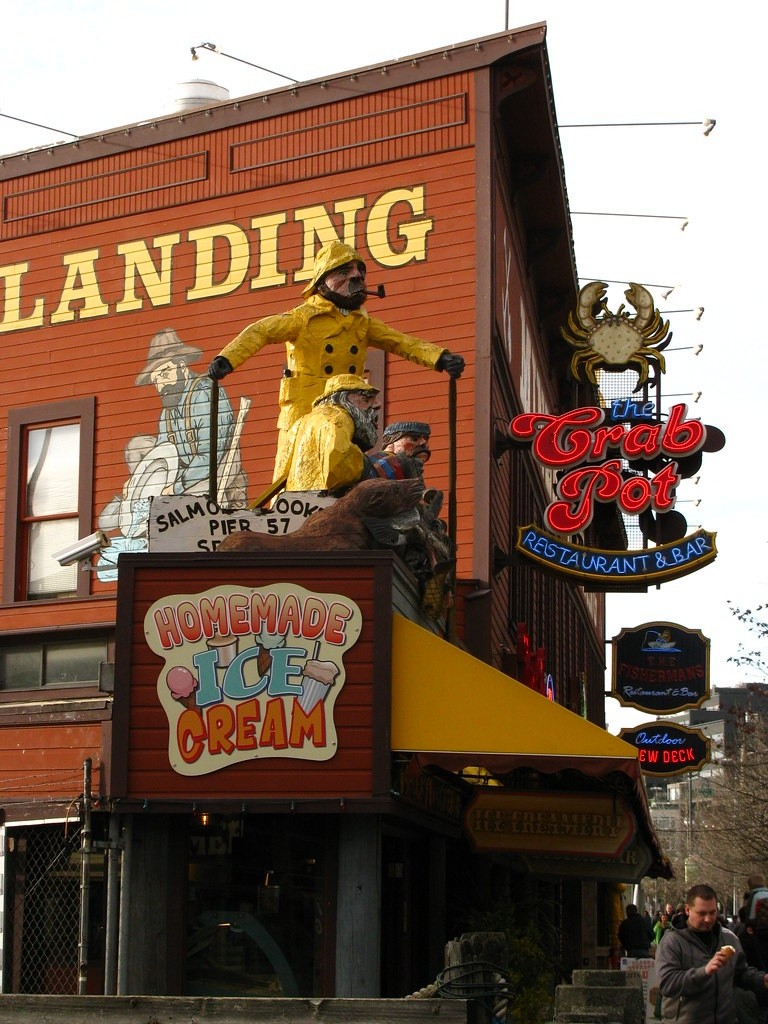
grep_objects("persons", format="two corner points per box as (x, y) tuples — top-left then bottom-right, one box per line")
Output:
(367, 421), (468, 621)
(643, 876), (768, 1024)
(654, 885), (768, 1024)
(277, 373), (383, 499)
(618, 904), (656, 958)
(207, 241), (466, 510)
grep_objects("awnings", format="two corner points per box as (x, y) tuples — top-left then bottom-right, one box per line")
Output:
(391, 612), (678, 881)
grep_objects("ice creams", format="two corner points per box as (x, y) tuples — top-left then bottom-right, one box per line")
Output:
(720, 945), (736, 961)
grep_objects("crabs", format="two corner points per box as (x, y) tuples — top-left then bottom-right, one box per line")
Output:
(559, 281), (674, 395)
